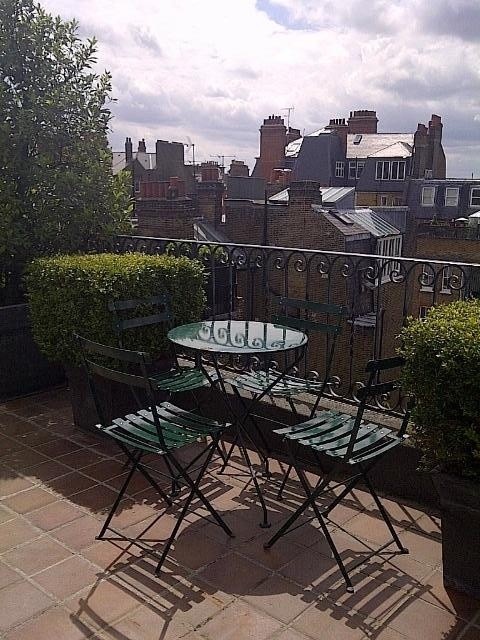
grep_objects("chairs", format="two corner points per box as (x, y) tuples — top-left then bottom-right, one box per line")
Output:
(71, 330), (233, 577)
(108, 294), (231, 497)
(263, 355), (411, 593)
(220, 295), (348, 500)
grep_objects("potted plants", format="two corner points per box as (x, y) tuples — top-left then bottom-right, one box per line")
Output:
(26, 251), (211, 438)
(0, 0), (138, 398)
(395, 292), (480, 620)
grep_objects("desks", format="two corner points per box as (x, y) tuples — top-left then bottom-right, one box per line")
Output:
(165, 320), (307, 527)
